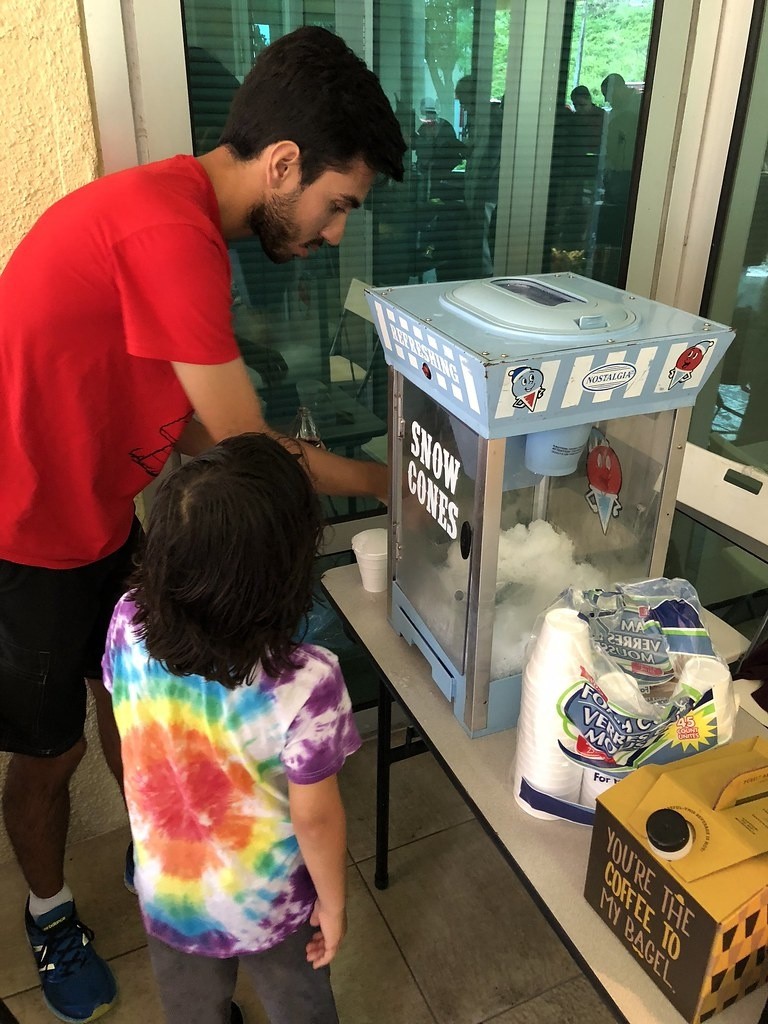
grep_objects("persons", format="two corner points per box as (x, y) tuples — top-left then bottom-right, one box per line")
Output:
(187, 47), (641, 388)
(0, 24), (425, 1023)
(100, 432), (364, 1024)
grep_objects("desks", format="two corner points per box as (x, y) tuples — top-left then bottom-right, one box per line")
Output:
(318, 562), (768, 1024)
(270, 341), (388, 514)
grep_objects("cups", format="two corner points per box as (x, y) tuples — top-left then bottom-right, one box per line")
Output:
(350, 527), (387, 593)
(509, 607), (737, 825)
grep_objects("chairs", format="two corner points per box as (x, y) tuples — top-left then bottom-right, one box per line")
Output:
(328, 278), (382, 406)
(633, 442), (768, 688)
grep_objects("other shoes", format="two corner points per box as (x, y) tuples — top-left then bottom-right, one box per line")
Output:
(229, 1000), (244, 1024)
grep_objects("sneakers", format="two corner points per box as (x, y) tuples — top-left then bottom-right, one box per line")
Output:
(124, 840), (140, 895)
(23, 893), (118, 1023)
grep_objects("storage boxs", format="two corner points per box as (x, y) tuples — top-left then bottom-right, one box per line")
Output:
(583, 736), (768, 1024)
